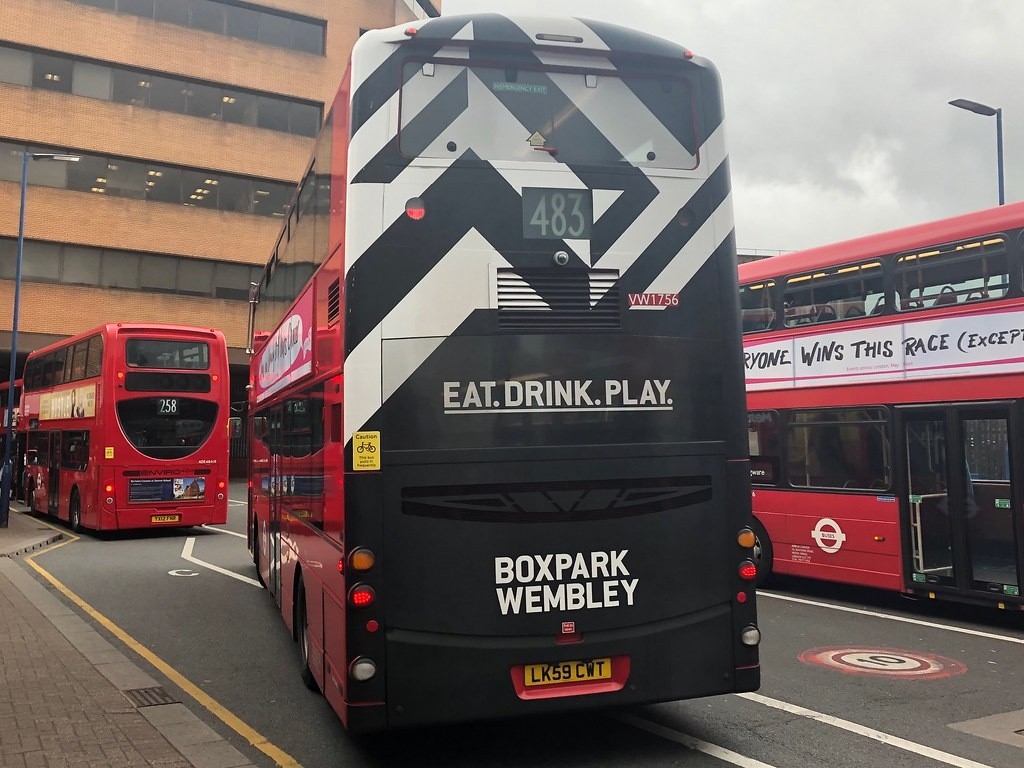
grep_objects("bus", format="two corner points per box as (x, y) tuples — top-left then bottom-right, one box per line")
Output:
(739, 195), (1024, 622)
(19, 320), (232, 533)
(0, 367), (99, 437)
(241, 14), (765, 748)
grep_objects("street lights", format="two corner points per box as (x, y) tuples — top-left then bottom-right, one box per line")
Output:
(949, 95), (1006, 206)
(0, 149), (83, 528)
